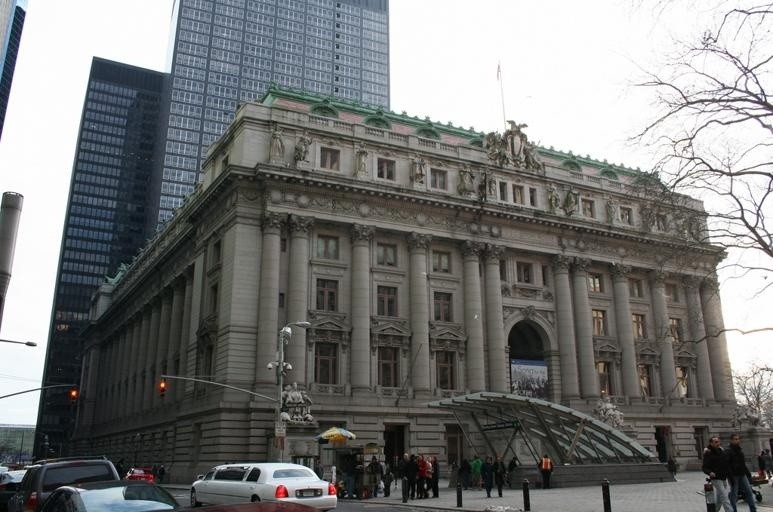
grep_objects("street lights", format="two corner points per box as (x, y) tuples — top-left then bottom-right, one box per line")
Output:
(266, 321), (312, 464)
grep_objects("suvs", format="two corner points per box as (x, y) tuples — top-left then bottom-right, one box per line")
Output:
(7, 458), (121, 512)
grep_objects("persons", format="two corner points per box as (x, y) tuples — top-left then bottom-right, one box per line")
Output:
(701, 435), (773, 512)
(315, 461), (324, 479)
(115, 458), (124, 477)
(397, 454), (439, 503)
(342, 457), (357, 498)
(159, 464), (165, 482)
(449, 455), (518, 497)
(366, 456), (383, 497)
(151, 464), (157, 478)
(381, 468), (394, 497)
(538, 454), (553, 489)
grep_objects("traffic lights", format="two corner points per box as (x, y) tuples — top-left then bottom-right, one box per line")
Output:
(159, 378), (167, 399)
(70, 387), (77, 407)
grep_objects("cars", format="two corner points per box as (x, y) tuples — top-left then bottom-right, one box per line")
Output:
(190, 461), (339, 511)
(123, 466), (155, 485)
(40, 478), (182, 512)
(0, 470), (28, 512)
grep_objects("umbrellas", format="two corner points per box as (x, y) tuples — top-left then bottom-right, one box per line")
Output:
(314, 427), (357, 444)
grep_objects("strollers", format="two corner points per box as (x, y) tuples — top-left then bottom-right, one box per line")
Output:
(727, 463), (769, 503)
